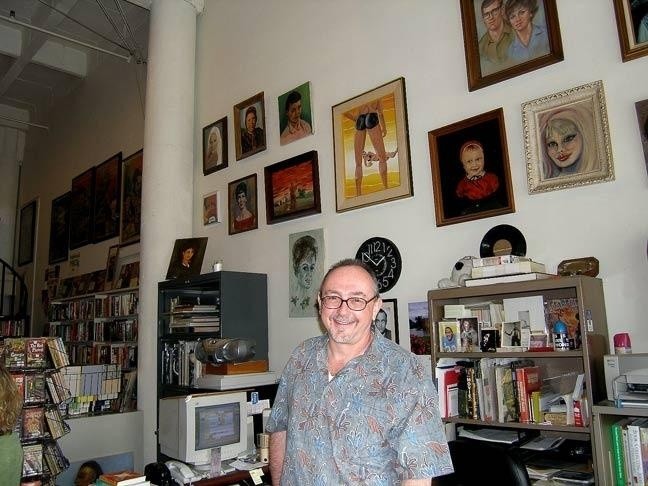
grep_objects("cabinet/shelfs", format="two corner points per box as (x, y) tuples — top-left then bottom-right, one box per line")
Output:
(46, 286), (139, 400)
(593, 399), (647, 485)
(157, 271), (269, 398)
(427, 275), (611, 484)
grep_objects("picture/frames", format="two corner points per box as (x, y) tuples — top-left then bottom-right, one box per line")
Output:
(460, 0), (564, 92)
(521, 80), (615, 195)
(191, 466), (270, 486)
(427, 107), (515, 227)
(376, 299), (399, 345)
(613, 0), (648, 174)
(18, 148), (143, 291)
(331, 77), (414, 213)
(203, 82), (321, 236)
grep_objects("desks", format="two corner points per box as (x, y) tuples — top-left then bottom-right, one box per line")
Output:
(191, 466), (270, 486)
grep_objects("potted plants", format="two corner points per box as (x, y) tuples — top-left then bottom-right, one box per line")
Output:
(460, 0), (564, 92)
(331, 77), (414, 213)
(18, 148), (143, 291)
(427, 107), (515, 227)
(203, 82), (321, 236)
(521, 80), (615, 195)
(208, 256), (224, 272)
(376, 299), (399, 345)
(613, 0), (648, 174)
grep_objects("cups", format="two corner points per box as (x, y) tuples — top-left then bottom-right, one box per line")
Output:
(319, 296), (377, 311)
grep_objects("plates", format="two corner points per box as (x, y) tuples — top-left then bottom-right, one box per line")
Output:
(157, 271), (269, 398)
(46, 286), (139, 400)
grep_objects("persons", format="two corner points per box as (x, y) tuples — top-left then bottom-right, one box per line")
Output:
(456, 140), (503, 202)
(287, 181), (303, 211)
(361, 146), (397, 168)
(265, 259), (455, 485)
(343, 99), (389, 195)
(505, 0), (549, 65)
(74, 461), (103, 486)
(537, 103), (601, 182)
(280, 90), (312, 147)
(204, 126), (224, 167)
(290, 235), (318, 295)
(374, 310), (392, 341)
(231, 182), (258, 231)
(168, 240), (201, 277)
(240, 106), (264, 154)
(478, 0), (514, 75)
(122, 168), (143, 238)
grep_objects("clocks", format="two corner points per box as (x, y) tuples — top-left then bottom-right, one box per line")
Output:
(353, 235), (404, 297)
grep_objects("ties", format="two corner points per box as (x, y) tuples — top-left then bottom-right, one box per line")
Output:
(159, 391), (248, 480)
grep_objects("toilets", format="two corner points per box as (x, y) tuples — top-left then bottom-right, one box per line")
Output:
(165, 460), (201, 484)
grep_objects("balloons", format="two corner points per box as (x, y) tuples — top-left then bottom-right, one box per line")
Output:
(353, 235), (404, 297)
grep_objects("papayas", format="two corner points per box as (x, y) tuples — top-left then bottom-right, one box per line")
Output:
(551, 321), (569, 352)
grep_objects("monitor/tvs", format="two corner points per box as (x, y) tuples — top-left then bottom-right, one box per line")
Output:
(0, 317), (70, 486)
(471, 261), (547, 278)
(46, 263), (220, 416)
(527, 464), (596, 486)
(434, 295), (591, 431)
(101, 469), (145, 486)
(96, 477), (151, 486)
(472, 255), (532, 267)
(466, 271), (559, 288)
(609, 415), (648, 486)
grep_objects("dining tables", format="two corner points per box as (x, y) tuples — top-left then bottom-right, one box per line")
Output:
(208, 256), (224, 272)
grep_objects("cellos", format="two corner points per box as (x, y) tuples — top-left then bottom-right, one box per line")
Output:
(602, 353), (648, 408)
